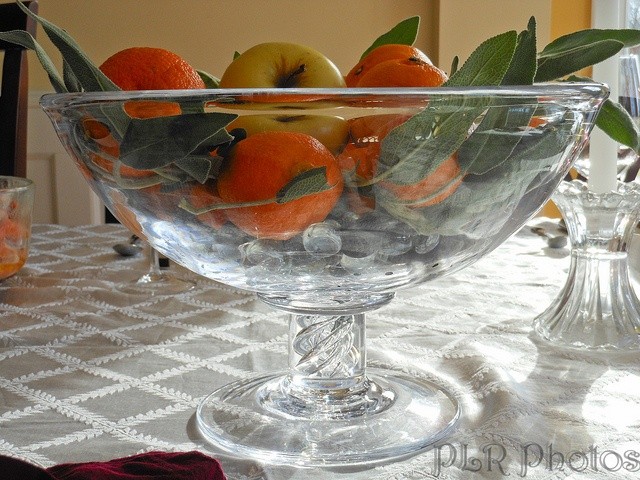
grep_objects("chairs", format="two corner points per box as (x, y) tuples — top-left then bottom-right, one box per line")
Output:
(0, 1), (37, 177)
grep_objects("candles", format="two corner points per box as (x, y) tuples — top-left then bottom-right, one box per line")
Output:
(589, 2), (620, 193)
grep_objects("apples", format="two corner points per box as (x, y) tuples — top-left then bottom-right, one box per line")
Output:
(221, 43), (347, 157)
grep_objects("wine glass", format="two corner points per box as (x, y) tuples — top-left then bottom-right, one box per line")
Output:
(38, 81), (610, 468)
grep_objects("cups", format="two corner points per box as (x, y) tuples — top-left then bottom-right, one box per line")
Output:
(0, 174), (32, 280)
